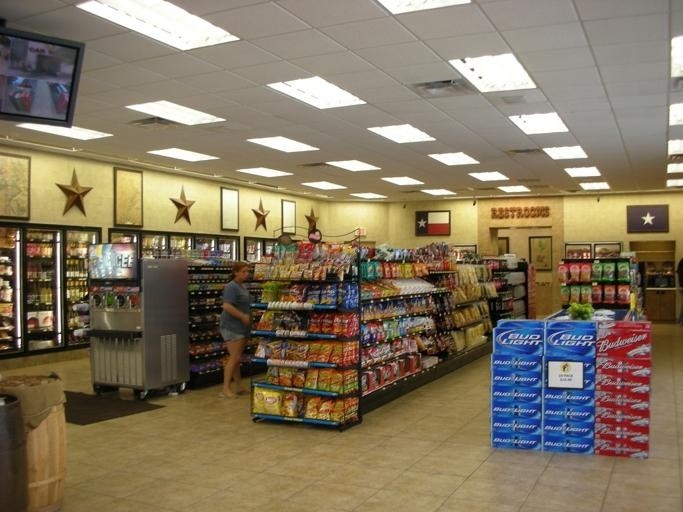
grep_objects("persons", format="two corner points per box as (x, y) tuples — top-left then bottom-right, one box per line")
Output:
(218, 261), (250, 398)
(674, 258), (683, 324)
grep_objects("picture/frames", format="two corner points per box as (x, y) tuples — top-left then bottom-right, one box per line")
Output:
(112, 166), (144, 229)
(497, 236), (508, 254)
(280, 199), (297, 236)
(0, 151), (32, 222)
(219, 186), (240, 231)
(528, 235), (552, 272)
(414, 210), (449, 236)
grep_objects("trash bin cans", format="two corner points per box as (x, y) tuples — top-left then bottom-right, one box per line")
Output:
(0, 393), (26, 512)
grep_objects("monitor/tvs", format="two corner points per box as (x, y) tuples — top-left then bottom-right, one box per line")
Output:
(0, 25), (85, 129)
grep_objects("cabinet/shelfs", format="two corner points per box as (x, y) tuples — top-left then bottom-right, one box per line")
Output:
(558, 257), (632, 310)
(359, 255), (528, 415)
(646, 291), (675, 321)
(247, 262), (363, 432)
(188, 260), (268, 391)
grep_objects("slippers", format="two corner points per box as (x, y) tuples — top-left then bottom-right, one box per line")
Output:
(232, 387), (248, 394)
(219, 392), (238, 399)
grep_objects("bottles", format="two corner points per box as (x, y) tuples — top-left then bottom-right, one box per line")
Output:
(24, 235), (91, 305)
